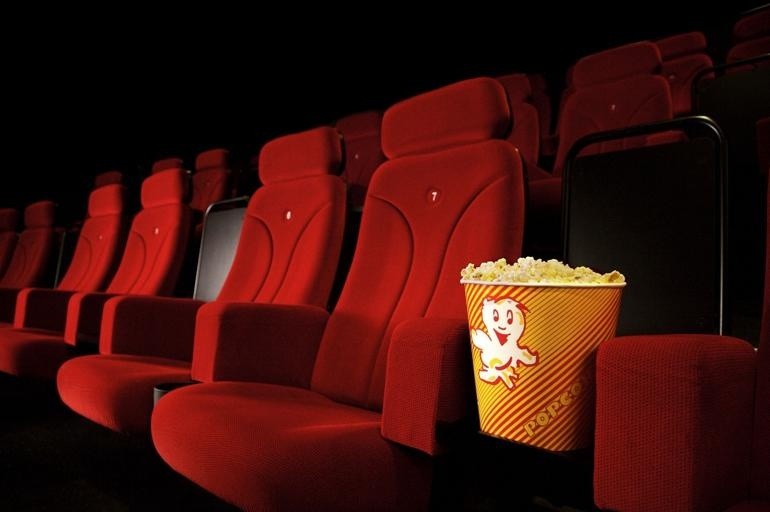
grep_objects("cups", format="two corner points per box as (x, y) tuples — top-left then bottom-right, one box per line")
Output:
(460, 278), (626, 452)
